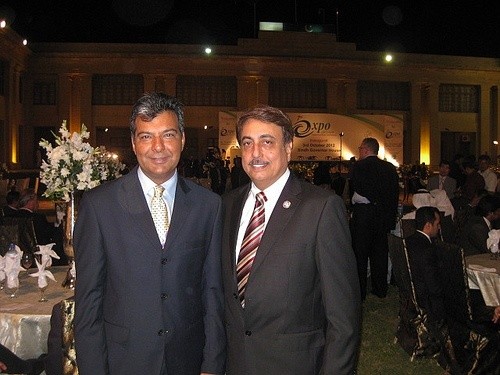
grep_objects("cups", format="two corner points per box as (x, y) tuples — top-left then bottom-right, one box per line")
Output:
(490, 244), (497, 260)
(42, 255), (50, 270)
(5, 274), (18, 298)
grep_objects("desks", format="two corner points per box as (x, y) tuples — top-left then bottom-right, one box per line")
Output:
(0, 265), (76, 361)
(465, 254), (500, 315)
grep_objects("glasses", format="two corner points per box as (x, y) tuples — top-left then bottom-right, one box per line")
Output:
(358, 146), (362, 150)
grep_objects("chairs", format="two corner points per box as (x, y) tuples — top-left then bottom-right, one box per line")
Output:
(388, 165), (500, 375)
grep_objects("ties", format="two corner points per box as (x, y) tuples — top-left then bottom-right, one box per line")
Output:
(149, 184), (170, 249)
(236, 192), (267, 308)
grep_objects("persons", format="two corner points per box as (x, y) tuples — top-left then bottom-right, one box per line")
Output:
(348, 137), (401, 298)
(219, 104), (362, 375)
(404, 204), (469, 328)
(1, 187), (67, 261)
(73, 92), (227, 375)
(400, 153), (500, 259)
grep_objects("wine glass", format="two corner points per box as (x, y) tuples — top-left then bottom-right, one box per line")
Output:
(38, 276), (48, 302)
(22, 254), (33, 276)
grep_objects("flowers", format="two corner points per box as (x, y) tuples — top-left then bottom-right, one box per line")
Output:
(38, 120), (126, 203)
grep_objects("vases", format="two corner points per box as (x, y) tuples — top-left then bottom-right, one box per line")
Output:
(61, 191), (83, 291)
